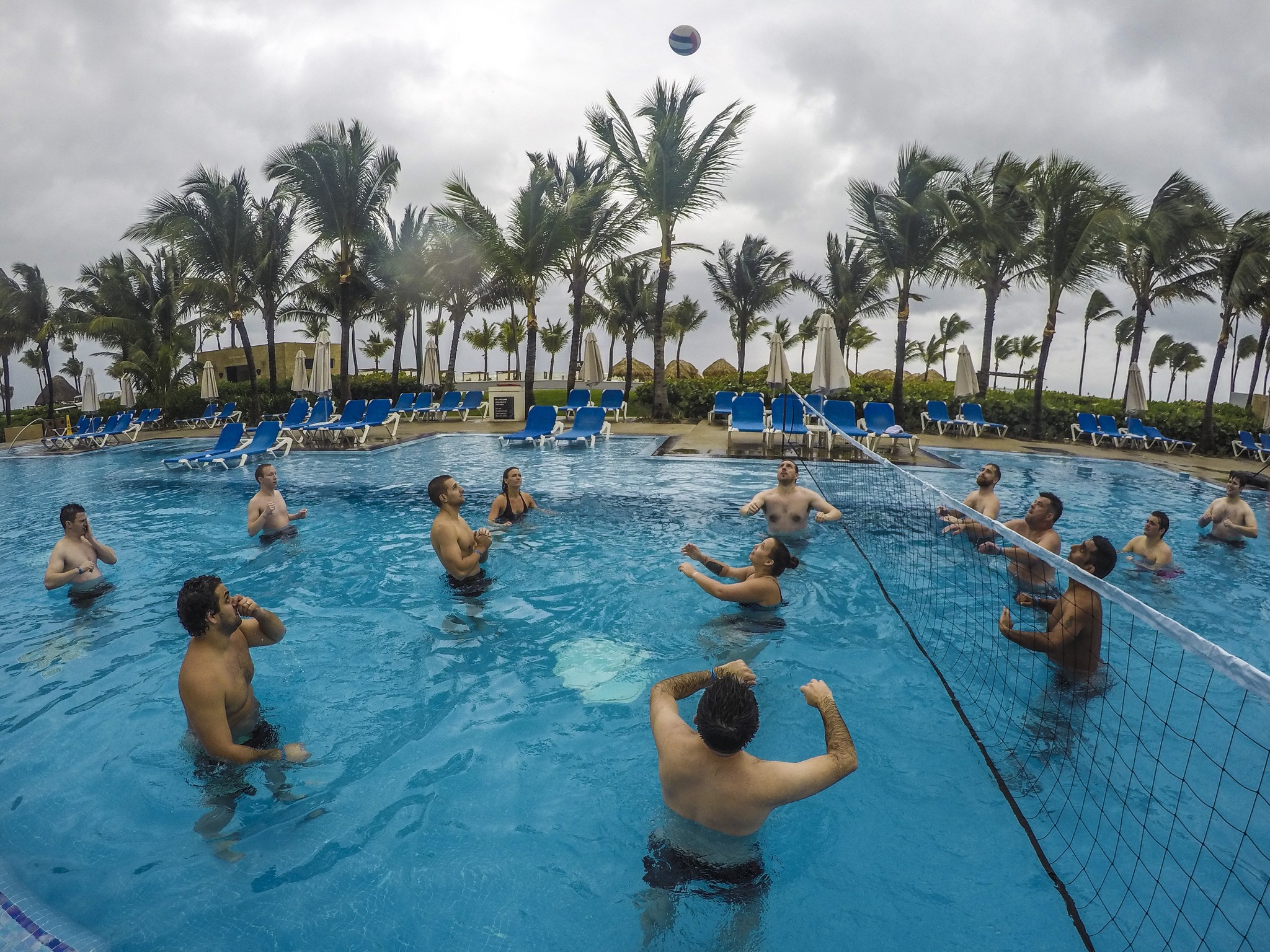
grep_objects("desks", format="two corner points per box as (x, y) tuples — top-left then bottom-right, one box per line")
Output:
(488, 387), (525, 422)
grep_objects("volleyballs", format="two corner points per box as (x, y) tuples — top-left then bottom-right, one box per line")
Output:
(668, 25), (701, 56)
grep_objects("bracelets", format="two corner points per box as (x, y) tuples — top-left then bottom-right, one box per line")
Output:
(961, 519), (964, 524)
(701, 555), (707, 564)
(474, 549), (483, 558)
(692, 572), (699, 580)
(708, 668), (716, 683)
(1032, 596), (1039, 608)
(78, 566), (83, 574)
(746, 510), (750, 515)
(997, 545), (1002, 556)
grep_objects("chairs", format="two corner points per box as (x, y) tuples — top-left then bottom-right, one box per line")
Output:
(40, 390), (488, 470)
(708, 391), (1008, 458)
(1070, 412), (1197, 455)
(496, 389), (628, 453)
(1232, 430), (1270, 463)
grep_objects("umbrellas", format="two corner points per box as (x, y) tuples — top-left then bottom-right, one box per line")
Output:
(766, 330), (792, 398)
(120, 374), (138, 415)
(81, 367), (101, 447)
(579, 328), (606, 407)
(953, 341), (980, 434)
(200, 361), (218, 417)
(419, 337), (440, 421)
(810, 310), (851, 425)
(291, 328), (332, 423)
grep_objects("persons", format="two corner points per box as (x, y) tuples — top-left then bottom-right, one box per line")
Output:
(43, 464), (539, 762)
(648, 463), (1258, 833)
